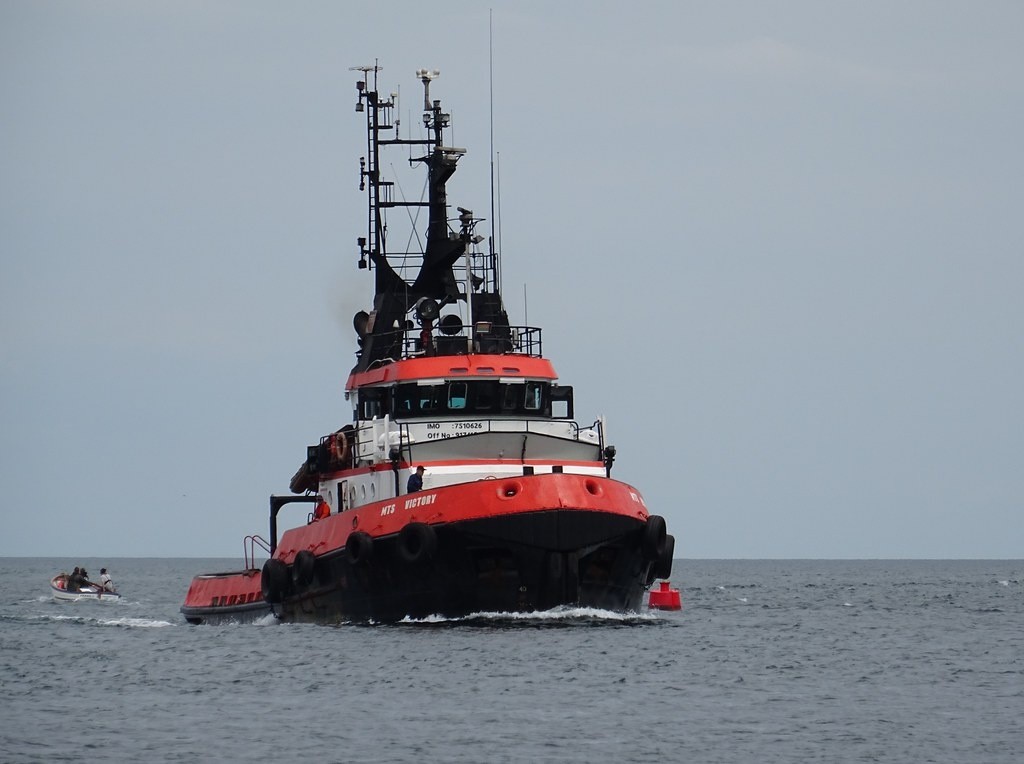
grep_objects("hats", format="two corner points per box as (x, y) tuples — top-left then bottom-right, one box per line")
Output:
(315, 494), (323, 499)
(417, 465), (425, 472)
(74, 567), (79, 572)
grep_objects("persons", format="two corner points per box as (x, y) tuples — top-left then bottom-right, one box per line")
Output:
(68, 567), (91, 592)
(100, 567), (113, 593)
(407, 465), (427, 493)
(314, 495), (331, 521)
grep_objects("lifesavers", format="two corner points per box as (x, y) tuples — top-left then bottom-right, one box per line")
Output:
(328, 434), (349, 459)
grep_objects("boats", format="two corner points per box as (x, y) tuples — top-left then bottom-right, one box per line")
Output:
(178, 53), (678, 630)
(49, 572), (121, 604)
